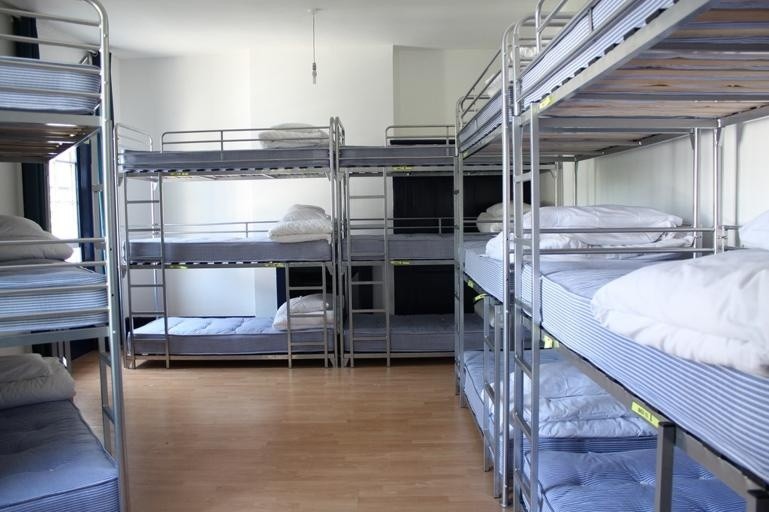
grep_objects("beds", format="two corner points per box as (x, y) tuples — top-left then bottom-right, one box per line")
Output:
(450, 22), (708, 510)
(331, 115), (565, 368)
(0, 2), (128, 511)
(114, 116), (338, 368)
(510, 1), (769, 510)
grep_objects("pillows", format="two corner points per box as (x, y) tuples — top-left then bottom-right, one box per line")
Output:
(0, 352), (53, 382)
(288, 294), (332, 311)
(282, 203), (326, 221)
(485, 200), (531, 219)
(520, 358), (607, 401)
(273, 122), (319, 134)
(0, 214), (42, 236)
(523, 202), (685, 245)
